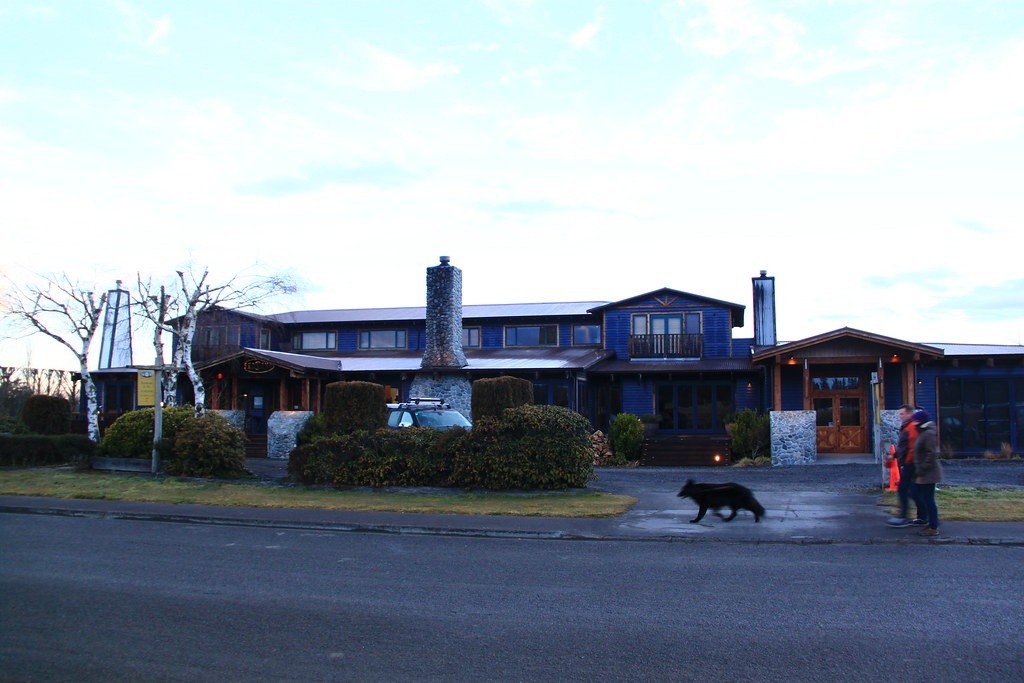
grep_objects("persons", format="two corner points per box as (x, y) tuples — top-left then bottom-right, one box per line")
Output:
(884, 404), (928, 528)
(910, 410), (942, 535)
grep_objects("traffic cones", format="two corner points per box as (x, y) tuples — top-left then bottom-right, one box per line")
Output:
(885, 444), (900, 491)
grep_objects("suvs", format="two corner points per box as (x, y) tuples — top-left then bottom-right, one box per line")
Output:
(385, 398), (473, 432)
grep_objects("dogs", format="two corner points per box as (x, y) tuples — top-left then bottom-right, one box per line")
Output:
(676, 478), (767, 523)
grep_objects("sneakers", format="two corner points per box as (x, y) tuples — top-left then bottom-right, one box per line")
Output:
(909, 517), (930, 526)
(884, 516), (912, 527)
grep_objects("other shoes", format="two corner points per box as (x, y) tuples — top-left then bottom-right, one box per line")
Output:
(917, 526), (941, 536)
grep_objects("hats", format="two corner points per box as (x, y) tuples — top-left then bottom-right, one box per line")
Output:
(911, 411), (930, 423)
